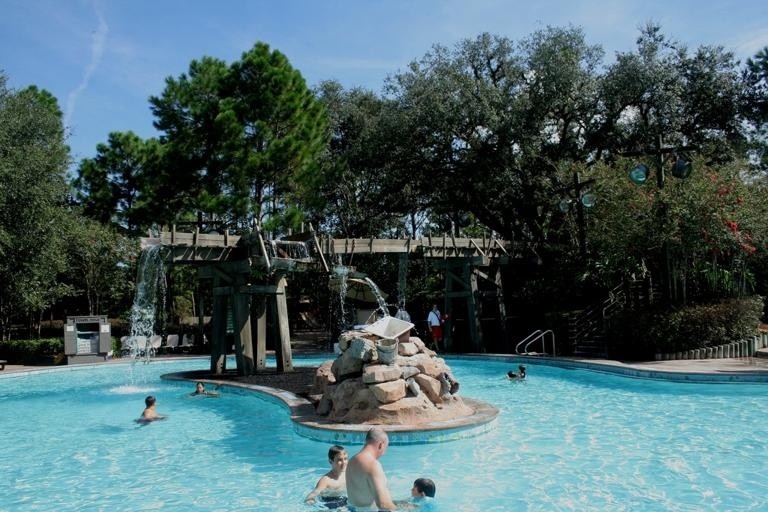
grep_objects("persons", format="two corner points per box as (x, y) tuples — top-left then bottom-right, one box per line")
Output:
(400, 478), (435, 509)
(304, 446), (348, 509)
(346, 428), (397, 512)
(427, 303), (442, 352)
(519, 365), (526, 378)
(191, 382), (221, 396)
(136, 396), (165, 425)
(508, 370), (517, 378)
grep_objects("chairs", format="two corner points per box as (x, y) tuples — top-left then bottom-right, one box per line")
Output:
(121, 334), (208, 355)
